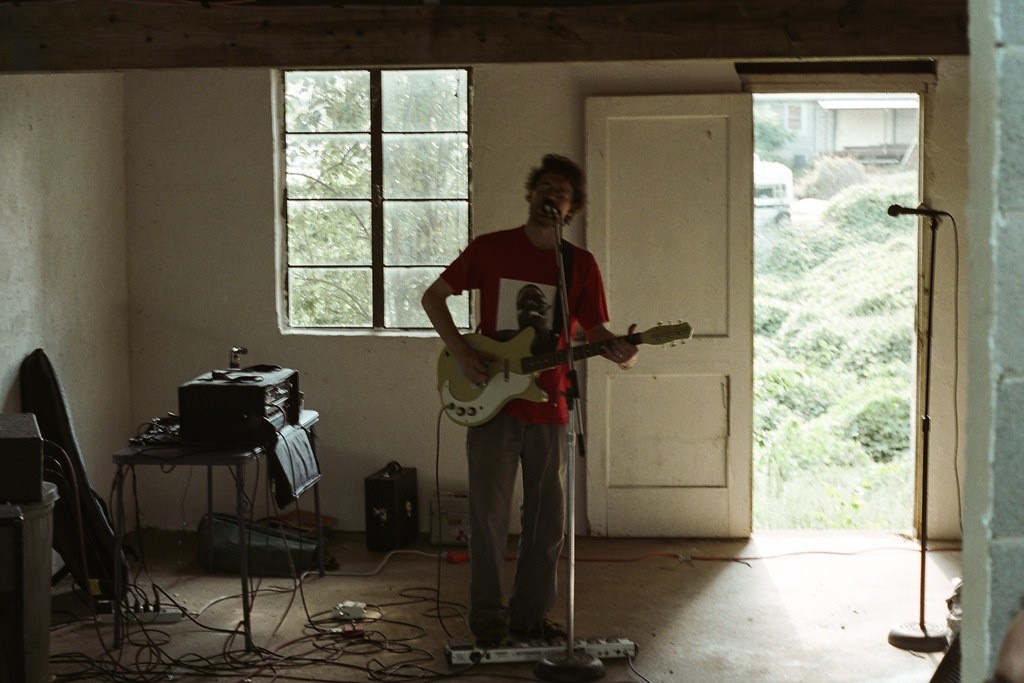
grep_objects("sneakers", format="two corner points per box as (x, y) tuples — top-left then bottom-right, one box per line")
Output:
(507, 612), (570, 642)
(476, 622), (507, 650)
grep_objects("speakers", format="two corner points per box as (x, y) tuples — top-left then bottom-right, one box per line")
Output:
(0, 413), (44, 503)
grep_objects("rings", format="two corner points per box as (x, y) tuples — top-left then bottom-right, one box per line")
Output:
(618, 353), (622, 358)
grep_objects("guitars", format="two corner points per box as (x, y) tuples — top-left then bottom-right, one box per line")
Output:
(438, 319), (695, 427)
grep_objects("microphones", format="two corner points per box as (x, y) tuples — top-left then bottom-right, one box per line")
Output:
(541, 200), (560, 215)
(887, 204), (950, 217)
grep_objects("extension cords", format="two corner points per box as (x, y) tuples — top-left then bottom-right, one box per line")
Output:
(119, 606), (182, 623)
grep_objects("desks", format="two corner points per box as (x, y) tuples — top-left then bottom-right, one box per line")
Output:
(113, 409), (320, 653)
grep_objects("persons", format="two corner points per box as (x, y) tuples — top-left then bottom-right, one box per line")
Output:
(421, 154), (639, 644)
